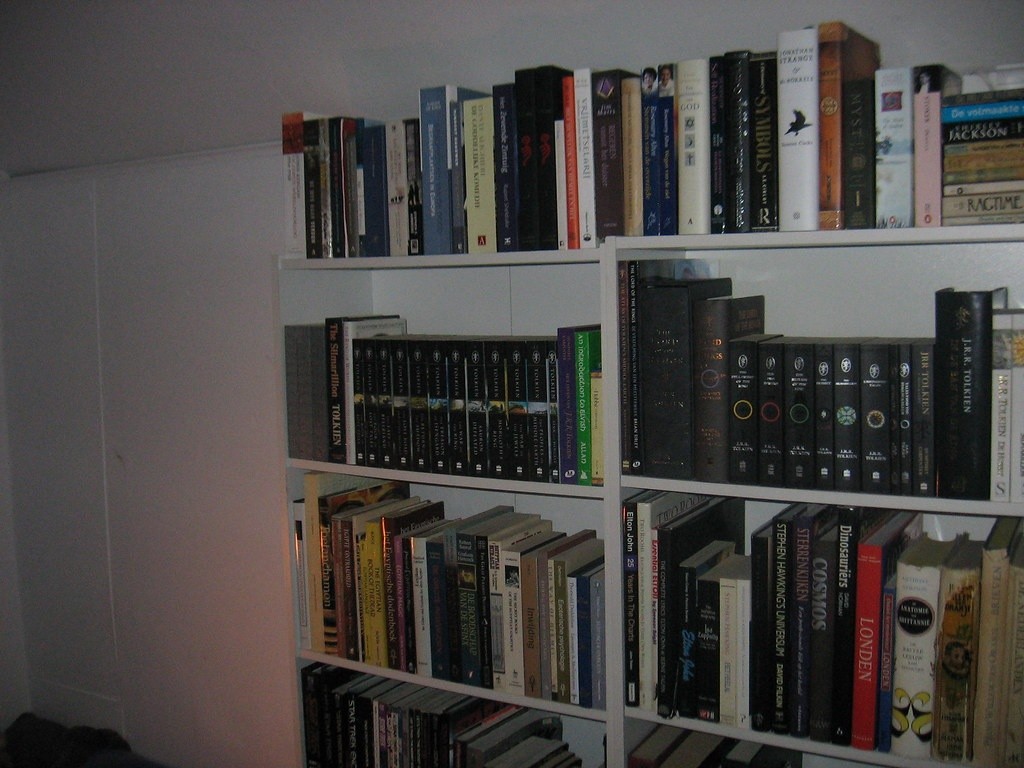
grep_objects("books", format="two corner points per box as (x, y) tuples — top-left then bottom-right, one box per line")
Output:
(618, 260), (1024, 501)
(282, 21), (1023, 258)
(301, 661), (606, 768)
(283, 313), (606, 487)
(621, 489), (1024, 768)
(292, 479), (606, 711)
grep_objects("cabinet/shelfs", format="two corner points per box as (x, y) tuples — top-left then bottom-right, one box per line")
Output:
(276, 220), (1024, 767)
